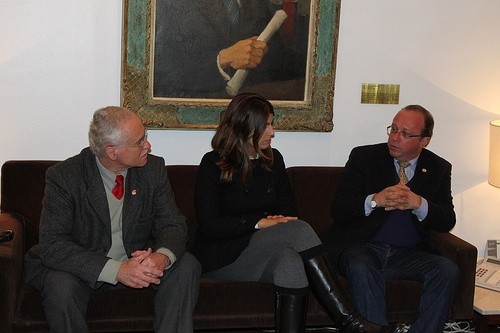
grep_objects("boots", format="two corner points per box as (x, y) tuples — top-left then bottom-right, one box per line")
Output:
(305, 250), (394, 333)
(275, 291), (307, 333)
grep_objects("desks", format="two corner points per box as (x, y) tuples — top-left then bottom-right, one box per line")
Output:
(473, 256), (500, 315)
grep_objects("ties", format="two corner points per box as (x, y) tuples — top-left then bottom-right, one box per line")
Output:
(397, 161), (410, 185)
(111, 174), (124, 200)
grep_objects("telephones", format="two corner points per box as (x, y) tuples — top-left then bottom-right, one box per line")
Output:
(475, 256), (500, 291)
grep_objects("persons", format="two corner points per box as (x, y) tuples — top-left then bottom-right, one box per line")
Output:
(324, 104), (460, 333)
(21, 106), (202, 333)
(192, 92), (405, 333)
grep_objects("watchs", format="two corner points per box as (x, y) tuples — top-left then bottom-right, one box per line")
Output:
(371, 197), (377, 209)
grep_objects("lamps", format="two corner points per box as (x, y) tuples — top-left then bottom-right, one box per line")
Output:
(488, 120), (500, 188)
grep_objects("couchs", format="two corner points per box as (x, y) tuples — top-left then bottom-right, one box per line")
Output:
(0, 160), (478, 333)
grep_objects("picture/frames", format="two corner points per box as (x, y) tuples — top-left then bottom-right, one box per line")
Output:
(120, 0), (341, 133)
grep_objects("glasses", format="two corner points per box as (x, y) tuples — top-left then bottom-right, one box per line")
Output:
(387, 125), (422, 140)
(113, 127), (148, 149)
